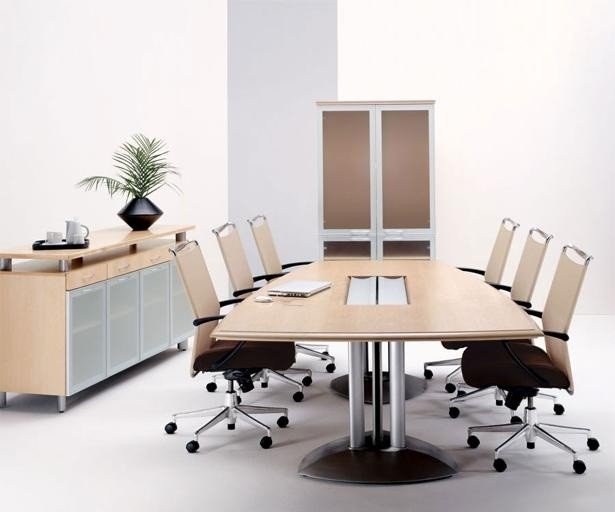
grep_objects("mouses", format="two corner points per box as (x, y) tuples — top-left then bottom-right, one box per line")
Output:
(254, 296), (273, 303)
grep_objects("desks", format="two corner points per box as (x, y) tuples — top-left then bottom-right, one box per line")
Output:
(210, 259), (544, 484)
(1, 226), (194, 411)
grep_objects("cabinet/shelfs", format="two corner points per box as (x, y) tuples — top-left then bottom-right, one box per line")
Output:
(316, 101), (437, 260)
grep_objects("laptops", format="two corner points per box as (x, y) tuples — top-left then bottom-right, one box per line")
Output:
(266, 279), (332, 298)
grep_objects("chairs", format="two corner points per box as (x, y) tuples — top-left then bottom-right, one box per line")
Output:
(205, 222), (311, 405)
(448, 228), (565, 426)
(425, 219), (519, 393)
(461, 244), (599, 474)
(247, 216), (336, 372)
(164, 240), (295, 452)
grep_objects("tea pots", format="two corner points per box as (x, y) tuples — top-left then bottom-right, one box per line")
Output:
(65, 220), (89, 244)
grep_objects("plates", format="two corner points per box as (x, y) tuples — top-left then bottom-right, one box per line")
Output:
(32, 239), (89, 250)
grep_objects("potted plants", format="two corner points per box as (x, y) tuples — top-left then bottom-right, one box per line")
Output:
(75, 133), (182, 231)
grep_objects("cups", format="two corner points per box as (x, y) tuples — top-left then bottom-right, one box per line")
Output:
(54, 233), (62, 242)
(74, 234), (85, 244)
(46, 231), (54, 242)
